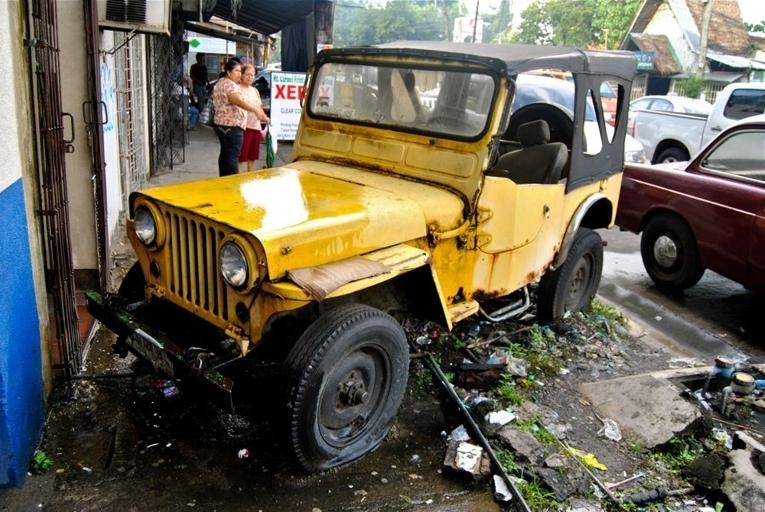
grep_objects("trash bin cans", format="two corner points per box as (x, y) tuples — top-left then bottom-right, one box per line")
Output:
(188, 106), (199, 130)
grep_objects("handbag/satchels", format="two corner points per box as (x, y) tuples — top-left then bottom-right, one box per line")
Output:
(198, 98), (214, 130)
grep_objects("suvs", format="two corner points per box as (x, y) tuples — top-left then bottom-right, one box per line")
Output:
(81, 37), (650, 478)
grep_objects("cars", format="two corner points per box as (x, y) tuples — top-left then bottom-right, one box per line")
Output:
(614, 115), (765, 302)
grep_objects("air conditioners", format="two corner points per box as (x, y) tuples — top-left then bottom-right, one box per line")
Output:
(97, 0), (170, 36)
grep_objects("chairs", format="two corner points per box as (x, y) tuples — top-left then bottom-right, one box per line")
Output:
(489, 121), (568, 183)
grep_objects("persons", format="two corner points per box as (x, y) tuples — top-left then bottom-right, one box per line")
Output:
(190, 52), (210, 111)
(212, 60), (272, 177)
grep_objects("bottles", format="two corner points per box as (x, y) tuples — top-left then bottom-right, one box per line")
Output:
(711, 355), (757, 395)
